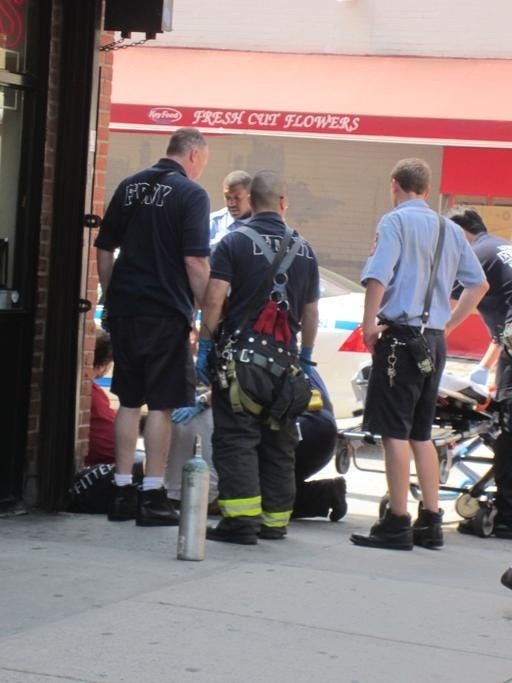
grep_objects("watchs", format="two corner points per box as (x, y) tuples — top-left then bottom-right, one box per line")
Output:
(197, 392), (211, 410)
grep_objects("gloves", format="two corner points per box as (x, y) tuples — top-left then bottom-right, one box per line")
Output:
(171, 395), (208, 425)
(471, 367), (490, 386)
(300, 343), (312, 376)
(195, 340), (213, 385)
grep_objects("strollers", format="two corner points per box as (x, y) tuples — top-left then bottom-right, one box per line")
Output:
(335, 359), (511, 538)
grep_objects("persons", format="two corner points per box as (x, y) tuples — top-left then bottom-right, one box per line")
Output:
(350, 157), (489, 552)
(209, 170), (253, 253)
(170, 365), (346, 522)
(195, 171), (318, 542)
(452, 209), (510, 540)
(86, 330), (119, 467)
(94, 128), (213, 525)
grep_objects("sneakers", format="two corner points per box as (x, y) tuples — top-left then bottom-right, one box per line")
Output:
(206, 519), (257, 544)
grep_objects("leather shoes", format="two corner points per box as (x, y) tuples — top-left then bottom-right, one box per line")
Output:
(330, 476), (347, 521)
(342, 506), (512, 549)
(108, 485), (181, 525)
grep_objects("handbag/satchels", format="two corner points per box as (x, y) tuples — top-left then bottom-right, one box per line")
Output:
(408, 329), (438, 381)
(273, 378), (316, 424)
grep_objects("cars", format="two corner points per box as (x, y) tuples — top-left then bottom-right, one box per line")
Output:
(305, 254), (380, 427)
